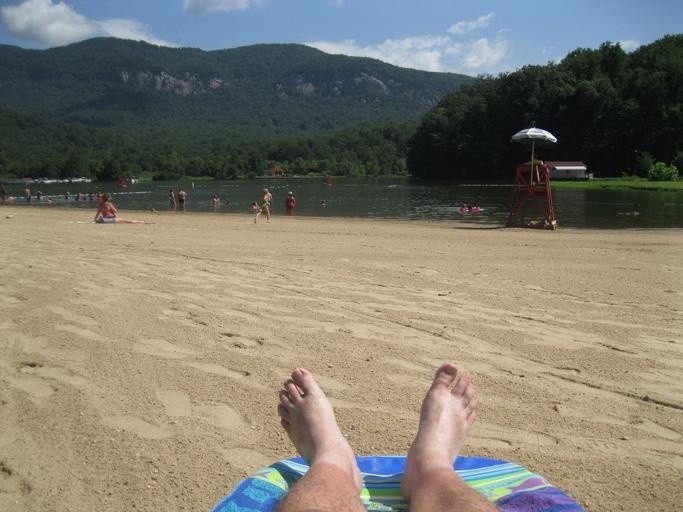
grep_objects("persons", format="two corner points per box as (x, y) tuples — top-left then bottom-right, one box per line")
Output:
(460, 203), (481, 215)
(286, 192), (295, 210)
(0, 175), (130, 222)
(213, 193), (232, 210)
(276, 362), (498, 512)
(169, 189), (177, 212)
(321, 199), (326, 206)
(249, 189), (273, 217)
(178, 188), (188, 211)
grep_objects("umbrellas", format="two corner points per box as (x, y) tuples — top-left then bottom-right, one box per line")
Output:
(511, 126), (557, 185)
